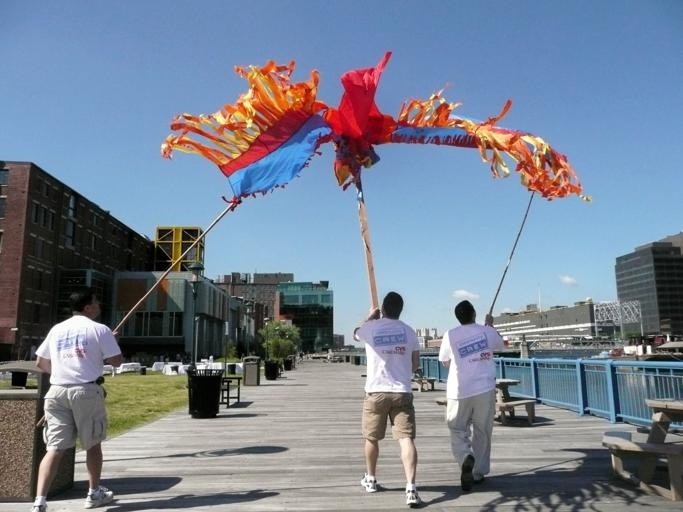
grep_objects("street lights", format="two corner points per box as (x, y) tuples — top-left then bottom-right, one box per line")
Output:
(187, 261), (289, 378)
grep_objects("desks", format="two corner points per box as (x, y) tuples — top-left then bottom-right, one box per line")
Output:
(493, 377), (521, 417)
(638, 394), (683, 484)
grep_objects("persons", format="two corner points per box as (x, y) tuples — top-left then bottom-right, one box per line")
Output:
(354, 292), (421, 505)
(33, 289), (124, 512)
(438, 300), (505, 491)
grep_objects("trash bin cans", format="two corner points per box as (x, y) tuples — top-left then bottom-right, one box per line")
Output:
(141, 368), (146, 375)
(228, 364), (236, 374)
(355, 356), (360, 365)
(0, 360), (75, 502)
(346, 356), (350, 362)
(264, 360), (279, 380)
(185, 369), (225, 419)
(170, 366), (178, 374)
(242, 356), (261, 386)
(283, 355), (296, 370)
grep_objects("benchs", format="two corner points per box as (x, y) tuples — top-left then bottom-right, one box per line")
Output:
(496, 398), (540, 424)
(411, 376), (436, 392)
(185, 361), (243, 408)
(603, 424), (683, 501)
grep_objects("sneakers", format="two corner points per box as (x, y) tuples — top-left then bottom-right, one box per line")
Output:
(405, 490), (421, 505)
(32, 503), (47, 511)
(461, 455), (474, 490)
(361, 473), (377, 493)
(85, 486), (115, 507)
